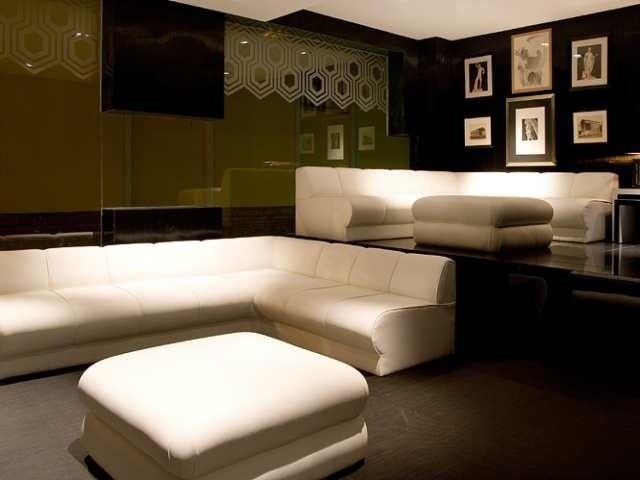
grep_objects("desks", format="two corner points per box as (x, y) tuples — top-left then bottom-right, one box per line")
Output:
(611, 188), (640, 244)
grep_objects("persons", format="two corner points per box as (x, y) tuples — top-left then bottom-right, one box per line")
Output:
(472, 63), (486, 90)
(583, 47), (596, 80)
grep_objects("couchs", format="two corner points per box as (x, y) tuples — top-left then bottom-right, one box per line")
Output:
(294, 165), (619, 242)
(0, 232), (458, 383)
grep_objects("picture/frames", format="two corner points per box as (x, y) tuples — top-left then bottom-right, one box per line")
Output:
(504, 93), (557, 168)
(461, 53), (495, 102)
(511, 28), (553, 94)
(461, 114), (495, 150)
(296, 95), (376, 162)
(568, 33), (611, 92)
(571, 108), (610, 146)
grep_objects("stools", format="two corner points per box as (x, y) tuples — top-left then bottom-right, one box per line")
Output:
(78, 331), (369, 480)
(413, 194), (553, 253)
